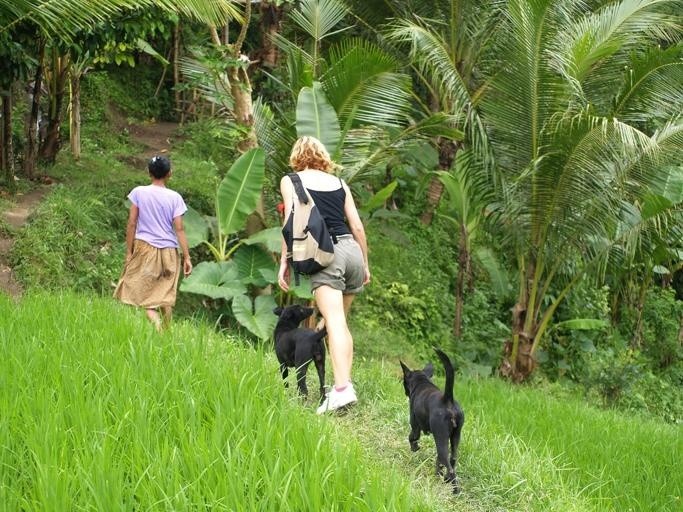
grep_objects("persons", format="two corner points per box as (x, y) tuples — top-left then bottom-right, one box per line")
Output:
(112, 156), (193, 334)
(277, 135), (371, 412)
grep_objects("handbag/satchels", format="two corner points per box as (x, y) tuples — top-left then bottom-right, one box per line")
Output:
(281, 172), (338, 274)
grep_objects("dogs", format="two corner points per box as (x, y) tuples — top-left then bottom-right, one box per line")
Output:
(271, 302), (327, 405)
(396, 348), (466, 493)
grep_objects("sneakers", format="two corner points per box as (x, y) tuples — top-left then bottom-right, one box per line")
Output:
(316, 382), (356, 415)
